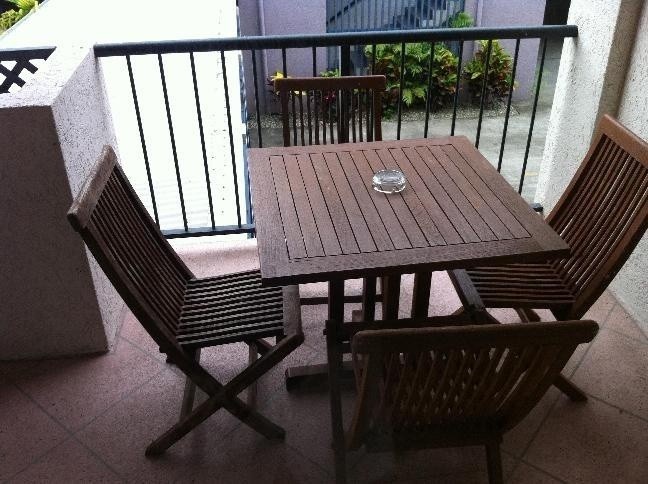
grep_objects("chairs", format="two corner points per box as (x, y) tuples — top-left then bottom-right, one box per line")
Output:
(67, 145), (304, 458)
(324, 304), (600, 484)
(445, 114), (648, 404)
(271, 75), (388, 304)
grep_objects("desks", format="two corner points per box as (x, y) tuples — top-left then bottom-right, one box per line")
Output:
(246, 134), (571, 391)
